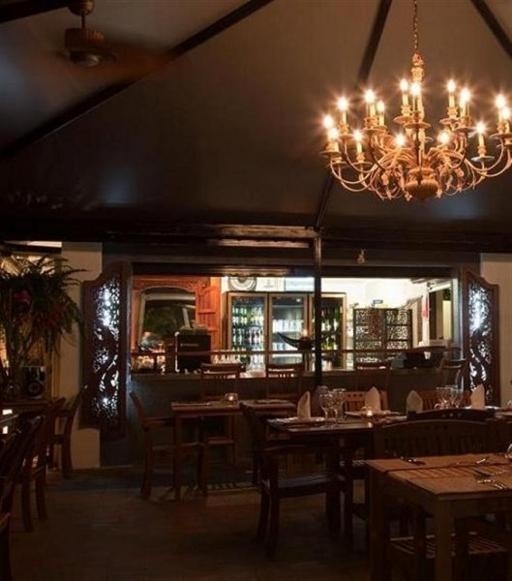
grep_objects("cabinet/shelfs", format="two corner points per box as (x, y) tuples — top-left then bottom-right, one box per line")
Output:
(222, 291), (347, 370)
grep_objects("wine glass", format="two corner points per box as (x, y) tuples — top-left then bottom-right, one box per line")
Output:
(436, 383), (464, 408)
(318, 383), (346, 430)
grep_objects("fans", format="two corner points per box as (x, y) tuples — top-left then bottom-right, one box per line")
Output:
(47, 0), (115, 67)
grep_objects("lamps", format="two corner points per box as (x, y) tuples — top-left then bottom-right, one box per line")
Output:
(315, 0), (511, 205)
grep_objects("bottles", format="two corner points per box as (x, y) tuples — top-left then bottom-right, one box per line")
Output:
(231, 305), (265, 366)
(321, 306), (341, 360)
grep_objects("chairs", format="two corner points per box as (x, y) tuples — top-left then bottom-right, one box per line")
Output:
(0, 360), (89, 580)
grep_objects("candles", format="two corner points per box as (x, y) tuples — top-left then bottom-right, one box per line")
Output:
(320, 67), (510, 152)
